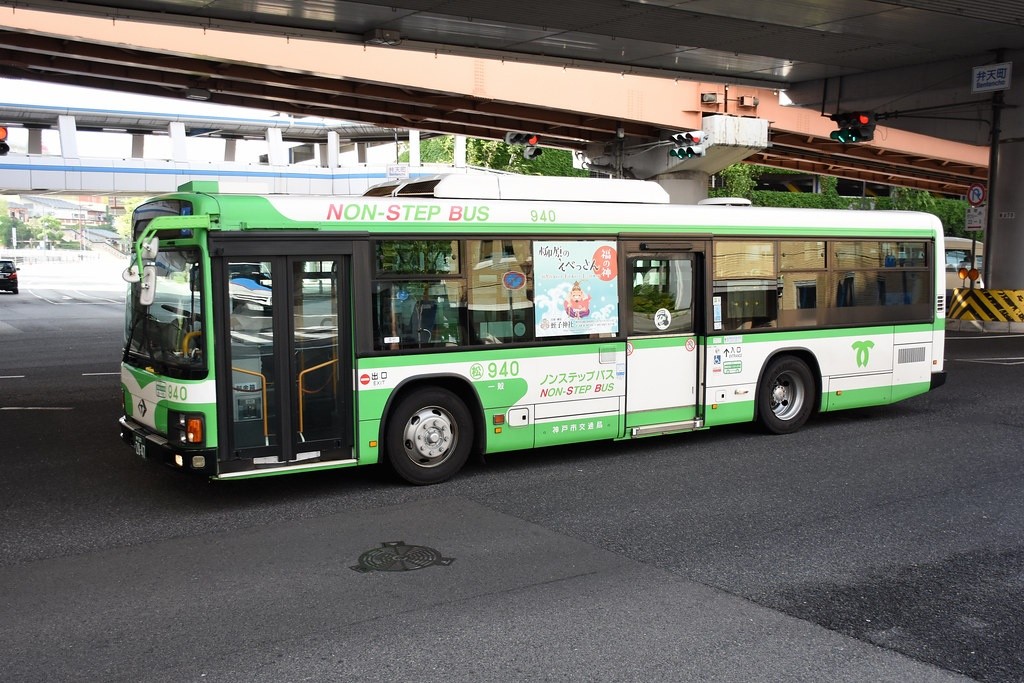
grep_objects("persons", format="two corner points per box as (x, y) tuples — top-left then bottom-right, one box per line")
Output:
(230, 300), (264, 345)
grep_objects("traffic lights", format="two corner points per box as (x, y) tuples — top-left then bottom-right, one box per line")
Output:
(668, 130), (707, 160)
(505, 132), (543, 160)
(829, 110), (877, 143)
(0, 126), (10, 157)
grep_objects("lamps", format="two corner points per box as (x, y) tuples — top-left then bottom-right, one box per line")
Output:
(616, 126), (625, 139)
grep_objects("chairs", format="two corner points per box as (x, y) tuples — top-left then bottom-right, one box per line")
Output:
(406, 300), (437, 343)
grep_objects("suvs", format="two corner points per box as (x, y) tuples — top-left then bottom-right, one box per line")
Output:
(0, 260), (21, 294)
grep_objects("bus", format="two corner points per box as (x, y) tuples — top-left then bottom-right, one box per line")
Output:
(943, 234), (984, 290)
(115, 172), (949, 487)
